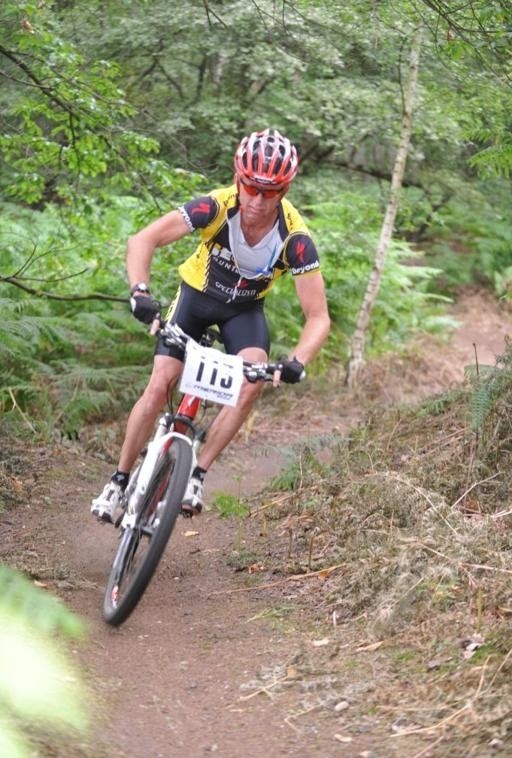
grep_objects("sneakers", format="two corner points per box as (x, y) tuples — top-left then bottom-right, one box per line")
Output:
(176, 466), (209, 519)
(89, 469), (130, 528)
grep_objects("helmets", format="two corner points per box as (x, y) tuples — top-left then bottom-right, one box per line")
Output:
(233, 128), (300, 183)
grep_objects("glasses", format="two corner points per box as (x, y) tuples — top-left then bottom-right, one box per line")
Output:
(236, 177), (289, 198)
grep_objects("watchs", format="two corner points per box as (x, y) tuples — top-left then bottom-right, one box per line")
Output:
(130, 282), (151, 296)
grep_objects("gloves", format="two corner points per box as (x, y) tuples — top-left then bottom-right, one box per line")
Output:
(274, 354), (307, 385)
(128, 293), (164, 335)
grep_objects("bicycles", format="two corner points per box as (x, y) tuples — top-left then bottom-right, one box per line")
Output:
(101, 308), (307, 630)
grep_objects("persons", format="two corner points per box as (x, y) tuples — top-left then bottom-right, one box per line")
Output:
(91, 128), (330, 524)
